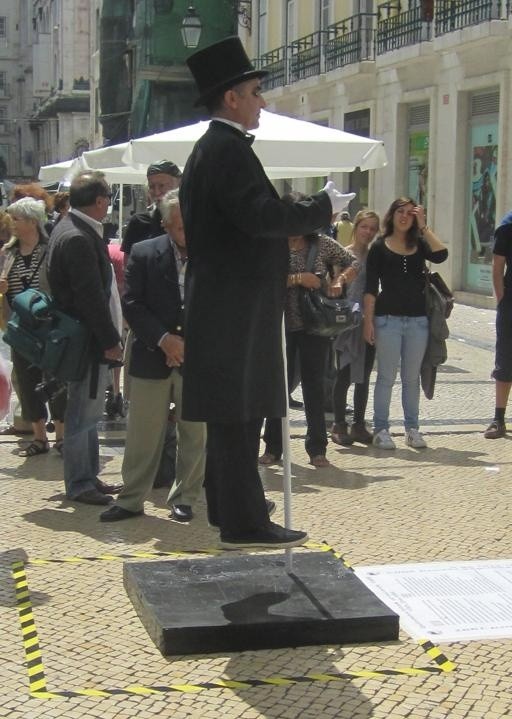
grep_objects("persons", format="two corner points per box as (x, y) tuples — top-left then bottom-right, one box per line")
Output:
(258, 183), (449, 467)
(484, 215), (511, 439)
(1, 158), (208, 523)
(178, 36), (357, 554)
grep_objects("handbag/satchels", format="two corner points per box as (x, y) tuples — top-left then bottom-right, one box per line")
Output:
(423, 271), (454, 320)
(299, 289), (354, 337)
(2, 288), (90, 389)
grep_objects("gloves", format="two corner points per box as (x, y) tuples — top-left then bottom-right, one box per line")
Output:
(323, 181), (357, 215)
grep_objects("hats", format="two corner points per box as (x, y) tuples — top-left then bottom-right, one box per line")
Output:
(185, 35), (269, 108)
(146, 159), (183, 178)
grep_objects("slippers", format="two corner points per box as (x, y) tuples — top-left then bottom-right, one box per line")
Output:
(0, 425), (34, 435)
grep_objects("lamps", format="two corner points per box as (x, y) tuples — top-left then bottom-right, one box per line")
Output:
(180, 1), (252, 49)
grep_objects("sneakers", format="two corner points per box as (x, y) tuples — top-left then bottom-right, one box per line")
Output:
(206, 498), (277, 531)
(258, 453), (276, 464)
(373, 428), (396, 450)
(291, 401), (302, 407)
(331, 421), (354, 446)
(344, 404), (353, 413)
(310, 455), (329, 467)
(483, 420), (506, 439)
(350, 421), (373, 445)
(219, 520), (310, 549)
(405, 428), (427, 448)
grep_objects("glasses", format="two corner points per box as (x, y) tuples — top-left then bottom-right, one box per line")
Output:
(106, 193), (112, 197)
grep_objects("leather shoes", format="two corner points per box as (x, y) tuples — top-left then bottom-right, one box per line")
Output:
(100, 506), (144, 522)
(73, 489), (113, 505)
(96, 481), (124, 494)
(170, 504), (194, 522)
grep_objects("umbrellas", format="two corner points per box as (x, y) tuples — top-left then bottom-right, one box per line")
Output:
(35, 144), (150, 240)
(78, 106), (389, 182)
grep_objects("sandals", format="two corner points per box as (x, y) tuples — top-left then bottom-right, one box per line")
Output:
(53, 439), (65, 460)
(18, 437), (50, 457)
